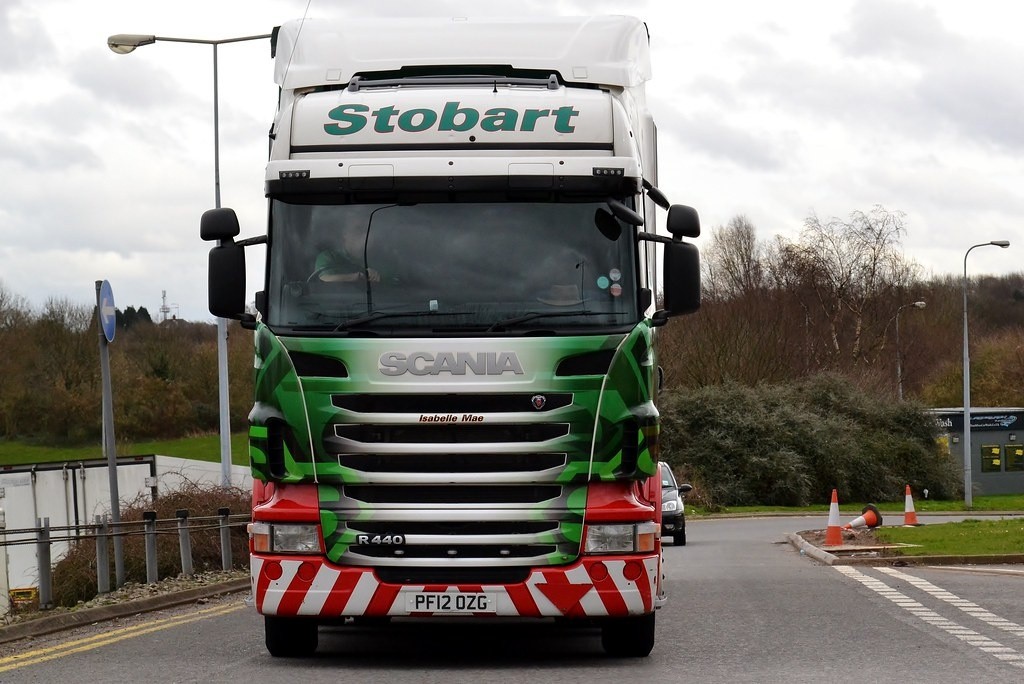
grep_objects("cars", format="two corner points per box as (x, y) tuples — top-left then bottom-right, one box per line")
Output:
(659, 461), (695, 547)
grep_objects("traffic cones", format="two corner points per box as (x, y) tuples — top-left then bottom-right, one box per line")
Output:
(901, 486), (920, 526)
(823, 485), (844, 549)
(845, 505), (885, 535)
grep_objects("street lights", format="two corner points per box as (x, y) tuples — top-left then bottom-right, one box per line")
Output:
(890, 296), (926, 406)
(102, 28), (233, 574)
(958, 230), (1009, 515)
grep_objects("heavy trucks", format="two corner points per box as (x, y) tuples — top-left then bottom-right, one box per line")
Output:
(202, 12), (700, 658)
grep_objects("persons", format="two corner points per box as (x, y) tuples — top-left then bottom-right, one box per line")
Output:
(315, 216), (381, 281)
